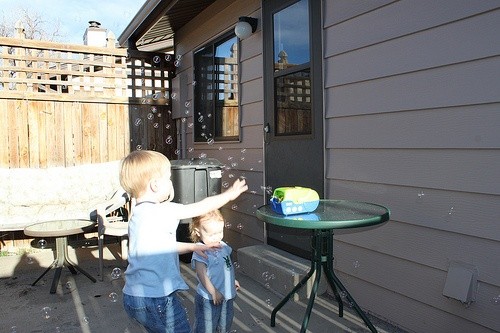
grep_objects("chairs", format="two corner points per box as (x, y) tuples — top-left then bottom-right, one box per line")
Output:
(24, 218), (97, 294)
(97, 193), (136, 280)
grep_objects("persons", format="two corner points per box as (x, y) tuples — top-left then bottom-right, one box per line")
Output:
(189, 208), (241, 333)
(119, 150), (249, 332)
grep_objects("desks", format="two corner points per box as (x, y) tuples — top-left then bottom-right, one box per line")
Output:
(256, 198), (390, 333)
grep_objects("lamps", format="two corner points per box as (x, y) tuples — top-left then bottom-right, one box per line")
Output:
(234, 15), (258, 39)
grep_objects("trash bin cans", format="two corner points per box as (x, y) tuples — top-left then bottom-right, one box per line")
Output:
(168, 157), (225, 264)
